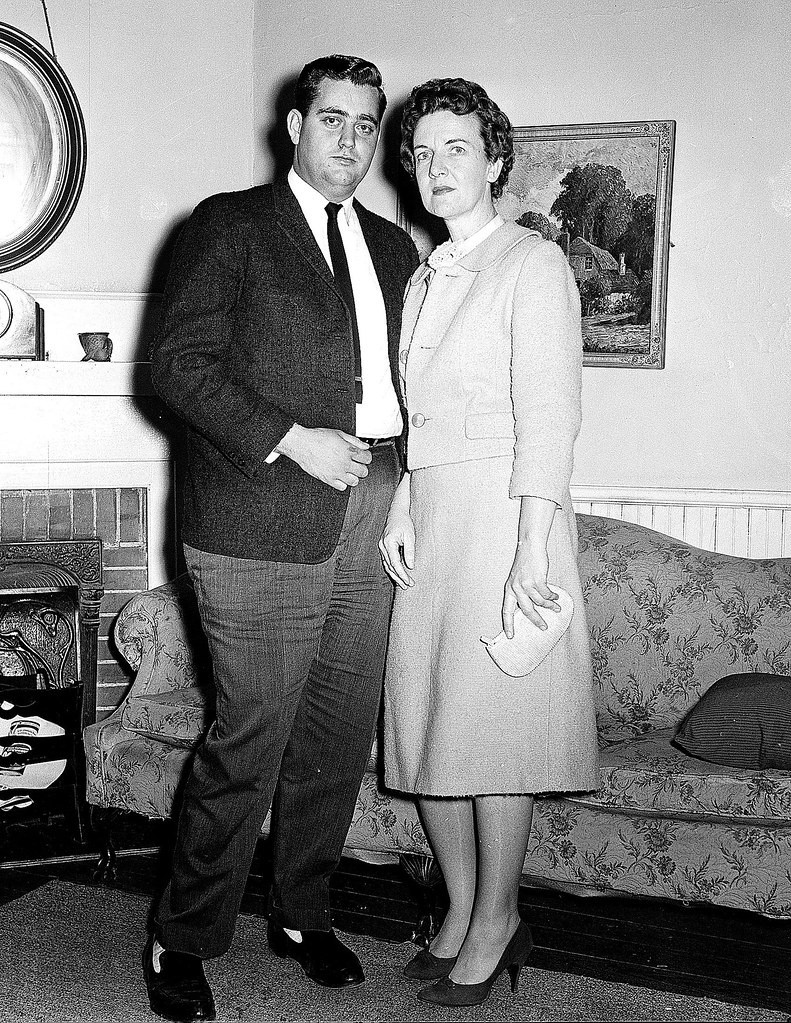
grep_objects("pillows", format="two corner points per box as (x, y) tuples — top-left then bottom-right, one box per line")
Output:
(672, 674), (791, 772)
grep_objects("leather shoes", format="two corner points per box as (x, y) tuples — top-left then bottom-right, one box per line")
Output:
(268, 920), (366, 989)
(142, 936), (217, 1023)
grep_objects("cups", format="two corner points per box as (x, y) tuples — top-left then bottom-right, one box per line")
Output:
(77, 332), (113, 362)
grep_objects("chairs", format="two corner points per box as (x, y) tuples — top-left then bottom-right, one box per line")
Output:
(0, 679), (88, 875)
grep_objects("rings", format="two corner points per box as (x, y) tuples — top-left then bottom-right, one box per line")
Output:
(381, 559), (386, 562)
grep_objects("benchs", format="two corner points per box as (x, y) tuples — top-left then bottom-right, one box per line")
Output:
(80, 513), (791, 937)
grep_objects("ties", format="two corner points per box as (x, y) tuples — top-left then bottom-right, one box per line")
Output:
(323, 202), (364, 404)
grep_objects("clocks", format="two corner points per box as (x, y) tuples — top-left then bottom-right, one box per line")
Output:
(0, 280), (45, 361)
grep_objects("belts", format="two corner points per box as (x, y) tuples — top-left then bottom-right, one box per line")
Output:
(359, 436), (397, 447)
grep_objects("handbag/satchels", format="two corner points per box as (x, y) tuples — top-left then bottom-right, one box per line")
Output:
(479, 583), (574, 676)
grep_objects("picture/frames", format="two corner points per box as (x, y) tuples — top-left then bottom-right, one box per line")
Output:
(0, 19), (88, 275)
(394, 118), (677, 372)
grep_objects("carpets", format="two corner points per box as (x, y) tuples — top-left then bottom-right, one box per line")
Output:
(0, 881), (791, 1023)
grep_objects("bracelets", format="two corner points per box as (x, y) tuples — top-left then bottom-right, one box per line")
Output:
(515, 539), (547, 550)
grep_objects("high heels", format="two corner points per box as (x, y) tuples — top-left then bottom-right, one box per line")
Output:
(418, 918), (531, 1006)
(401, 946), (459, 982)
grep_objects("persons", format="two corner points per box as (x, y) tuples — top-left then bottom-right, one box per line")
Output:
(375, 77), (604, 1007)
(140, 53), (420, 1023)
